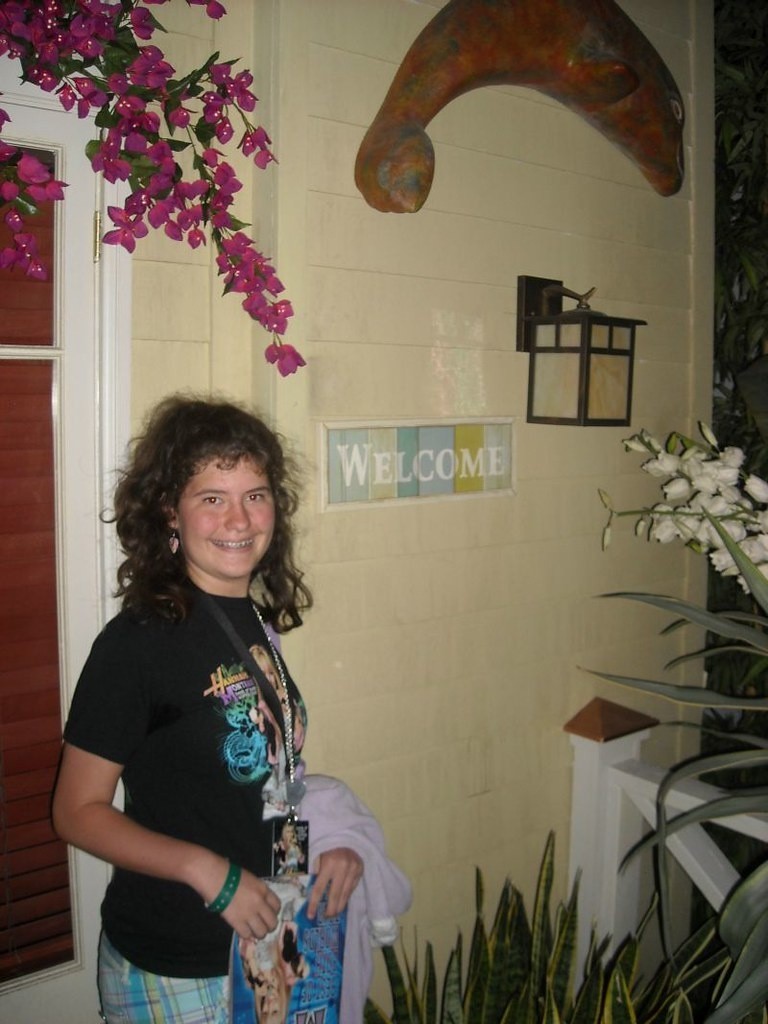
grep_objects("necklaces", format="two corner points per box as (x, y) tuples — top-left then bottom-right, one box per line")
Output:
(252, 603), (301, 824)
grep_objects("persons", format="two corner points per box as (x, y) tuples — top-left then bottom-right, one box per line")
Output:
(237, 920), (309, 1024)
(53, 396), (362, 1024)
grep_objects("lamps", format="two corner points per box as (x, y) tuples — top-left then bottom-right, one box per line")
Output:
(516, 275), (648, 427)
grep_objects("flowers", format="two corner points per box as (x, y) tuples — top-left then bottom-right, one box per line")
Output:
(0, 0), (307, 378)
(577, 422), (768, 1024)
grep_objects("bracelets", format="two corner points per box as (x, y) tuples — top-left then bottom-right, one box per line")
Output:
(205, 857), (242, 918)
